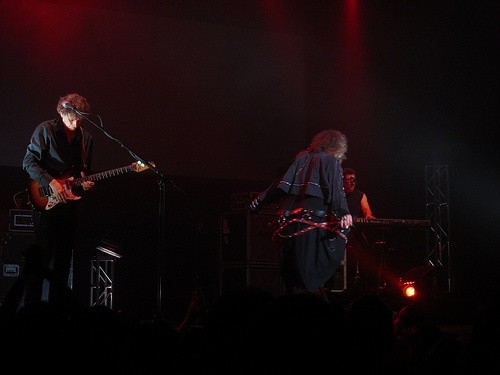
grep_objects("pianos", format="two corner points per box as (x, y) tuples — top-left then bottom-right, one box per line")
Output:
(353, 217), (431, 225)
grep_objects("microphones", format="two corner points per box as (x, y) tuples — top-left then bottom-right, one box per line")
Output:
(62, 101), (82, 115)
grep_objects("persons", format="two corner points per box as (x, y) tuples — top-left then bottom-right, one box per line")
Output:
(22, 93), (99, 318)
(262, 130), (352, 298)
(340, 170), (377, 242)
(0, 271), (500, 375)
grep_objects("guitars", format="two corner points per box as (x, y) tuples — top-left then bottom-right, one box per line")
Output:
(25, 160), (157, 212)
(271, 207), (354, 241)
